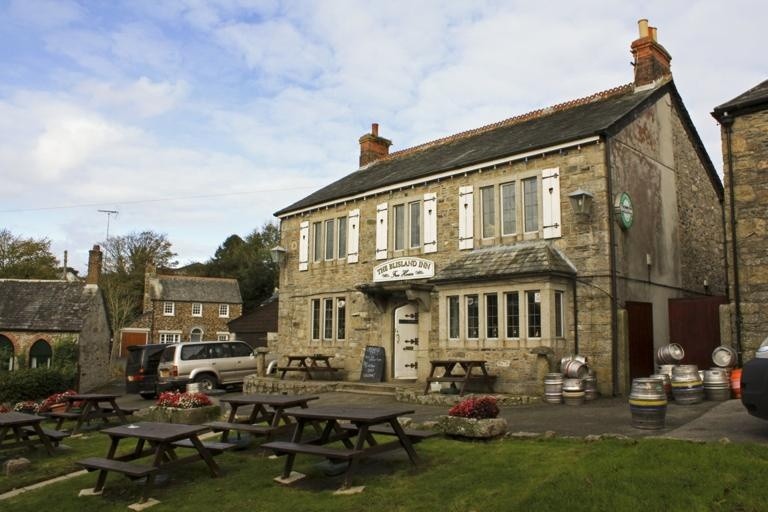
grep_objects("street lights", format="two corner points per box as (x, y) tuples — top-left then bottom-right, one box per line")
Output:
(98, 209), (119, 274)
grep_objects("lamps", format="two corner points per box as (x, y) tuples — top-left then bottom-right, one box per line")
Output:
(268, 242), (288, 266)
(567, 186), (595, 226)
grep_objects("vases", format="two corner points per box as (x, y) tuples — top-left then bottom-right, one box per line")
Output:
(51, 400), (84, 413)
(440, 415), (508, 437)
(149, 405), (221, 426)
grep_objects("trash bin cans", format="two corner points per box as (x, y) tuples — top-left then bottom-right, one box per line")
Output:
(359, 345), (385, 382)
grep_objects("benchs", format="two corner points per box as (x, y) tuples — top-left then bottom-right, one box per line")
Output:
(340, 423), (438, 445)
(426, 377), (475, 383)
(17, 425), (72, 441)
(207, 420), (275, 437)
(452, 374), (496, 380)
(259, 440), (364, 469)
(147, 434), (238, 456)
(277, 367), (338, 372)
(72, 456), (159, 482)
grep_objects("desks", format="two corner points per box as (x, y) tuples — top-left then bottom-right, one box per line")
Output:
(423, 359), (492, 397)
(1, 410), (57, 463)
(219, 395), (320, 443)
(53, 394), (129, 433)
(280, 355), (336, 381)
(93, 420), (224, 503)
(277, 409), (424, 491)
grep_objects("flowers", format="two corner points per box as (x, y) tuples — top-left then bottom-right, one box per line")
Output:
(447, 396), (499, 418)
(157, 390), (212, 408)
(37, 390), (79, 412)
(14, 401), (39, 413)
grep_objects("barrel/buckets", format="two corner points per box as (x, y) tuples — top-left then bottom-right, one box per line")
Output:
(543, 354), (598, 408)
(629, 342), (743, 430)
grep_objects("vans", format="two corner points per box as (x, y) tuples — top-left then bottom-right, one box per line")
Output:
(125, 341), (276, 399)
